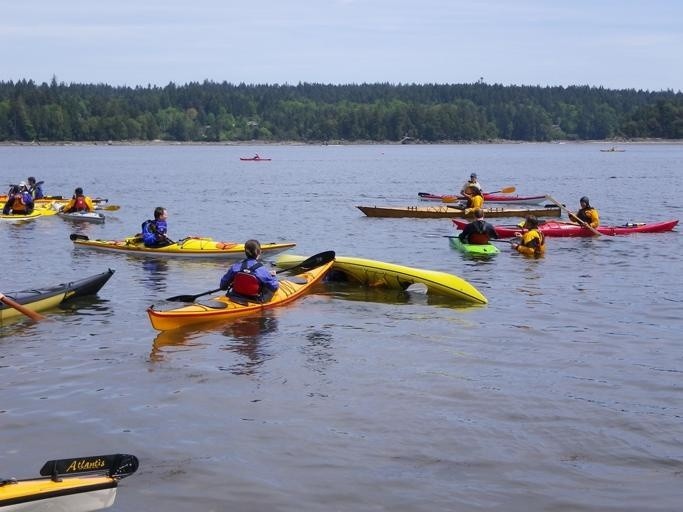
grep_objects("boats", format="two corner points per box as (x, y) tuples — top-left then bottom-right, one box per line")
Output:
(304, 280), (483, 312)
(0, 192), (121, 227)
(147, 283), (332, 364)
(72, 233), (299, 263)
(1, 269), (118, 322)
(269, 253), (491, 309)
(0, 451), (141, 511)
(0, 295), (111, 338)
(599, 148), (627, 152)
(239, 157), (272, 161)
(144, 251), (336, 336)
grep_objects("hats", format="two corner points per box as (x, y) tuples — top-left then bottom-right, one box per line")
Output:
(471, 173), (476, 177)
(19, 181), (26, 186)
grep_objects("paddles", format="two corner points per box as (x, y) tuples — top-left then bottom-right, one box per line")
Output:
(545, 193), (604, 236)
(165, 250), (335, 304)
(93, 205), (120, 211)
(441, 186), (515, 204)
(0, 291), (56, 327)
(441, 234), (521, 245)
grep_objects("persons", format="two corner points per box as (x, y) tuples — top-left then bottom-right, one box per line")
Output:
(511, 215), (544, 258)
(27, 177), (43, 201)
(461, 185), (483, 216)
(2, 184), (34, 216)
(566, 195), (600, 230)
(459, 208), (499, 245)
(141, 207), (171, 248)
(218, 240), (278, 299)
(18, 181), (29, 195)
(460, 173), (485, 199)
(61, 188), (94, 214)
